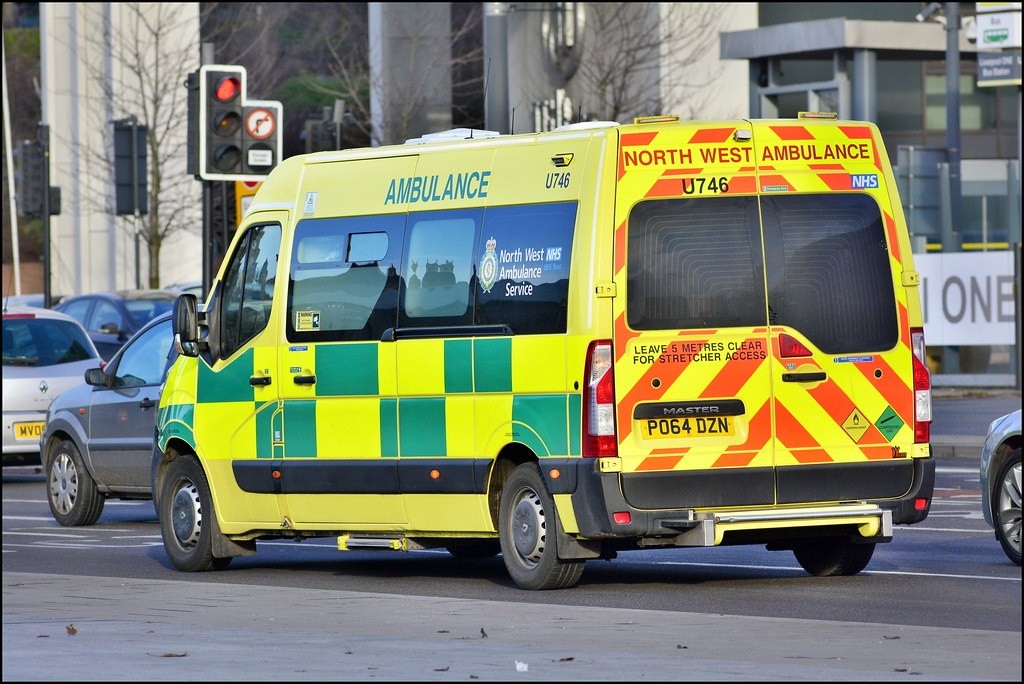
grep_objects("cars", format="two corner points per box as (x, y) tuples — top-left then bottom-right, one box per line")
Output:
(978, 409), (1024, 565)
(2, 293), (46, 308)
(2, 306), (109, 467)
(38, 304), (211, 528)
(165, 282), (205, 304)
(51, 291), (189, 364)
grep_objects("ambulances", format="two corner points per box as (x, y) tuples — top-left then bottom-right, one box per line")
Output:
(148, 112), (936, 590)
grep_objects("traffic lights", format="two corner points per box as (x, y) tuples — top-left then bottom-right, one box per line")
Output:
(11, 141), (41, 218)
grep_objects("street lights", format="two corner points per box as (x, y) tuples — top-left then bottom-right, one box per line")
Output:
(199, 64), (282, 182)
